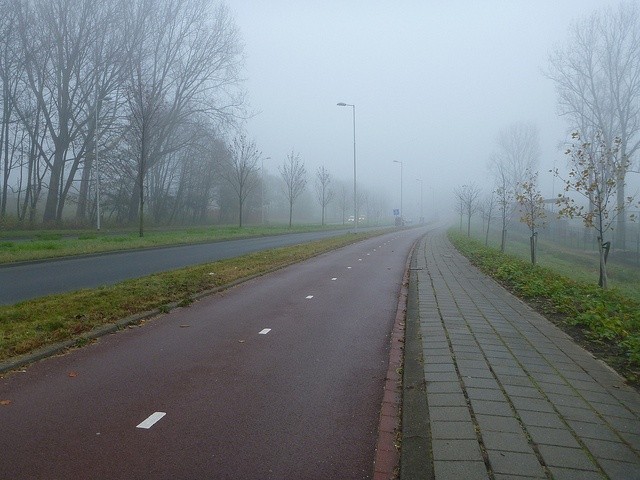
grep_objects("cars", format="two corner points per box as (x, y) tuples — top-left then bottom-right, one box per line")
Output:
(348, 213), (424, 227)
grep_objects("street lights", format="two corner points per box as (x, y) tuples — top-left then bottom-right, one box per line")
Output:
(337, 102), (358, 235)
(393, 159), (403, 227)
(95, 96), (113, 232)
(261, 156), (271, 224)
